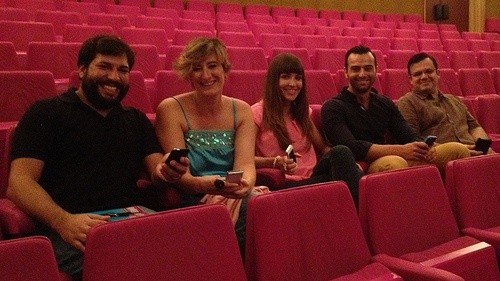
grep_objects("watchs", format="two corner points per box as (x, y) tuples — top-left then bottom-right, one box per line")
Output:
(215, 179), (223, 189)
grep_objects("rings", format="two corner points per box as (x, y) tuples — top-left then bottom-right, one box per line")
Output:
(283, 163), (287, 170)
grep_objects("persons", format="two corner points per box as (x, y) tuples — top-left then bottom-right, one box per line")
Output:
(5, 35), (190, 281)
(396, 52), (496, 156)
(250, 53), (365, 213)
(320, 45), (471, 174)
(155, 36), (271, 260)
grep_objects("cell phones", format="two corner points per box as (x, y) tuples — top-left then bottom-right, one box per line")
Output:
(424, 135), (437, 145)
(286, 145), (296, 165)
(475, 138), (492, 155)
(226, 169), (244, 186)
(165, 148), (190, 167)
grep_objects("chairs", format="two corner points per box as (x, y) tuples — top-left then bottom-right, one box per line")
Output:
(1, 0), (500, 281)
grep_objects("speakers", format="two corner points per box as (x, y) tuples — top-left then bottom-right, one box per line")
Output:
(435, 4), (449, 20)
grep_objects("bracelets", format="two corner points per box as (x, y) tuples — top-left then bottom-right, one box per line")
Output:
(273, 155), (280, 167)
